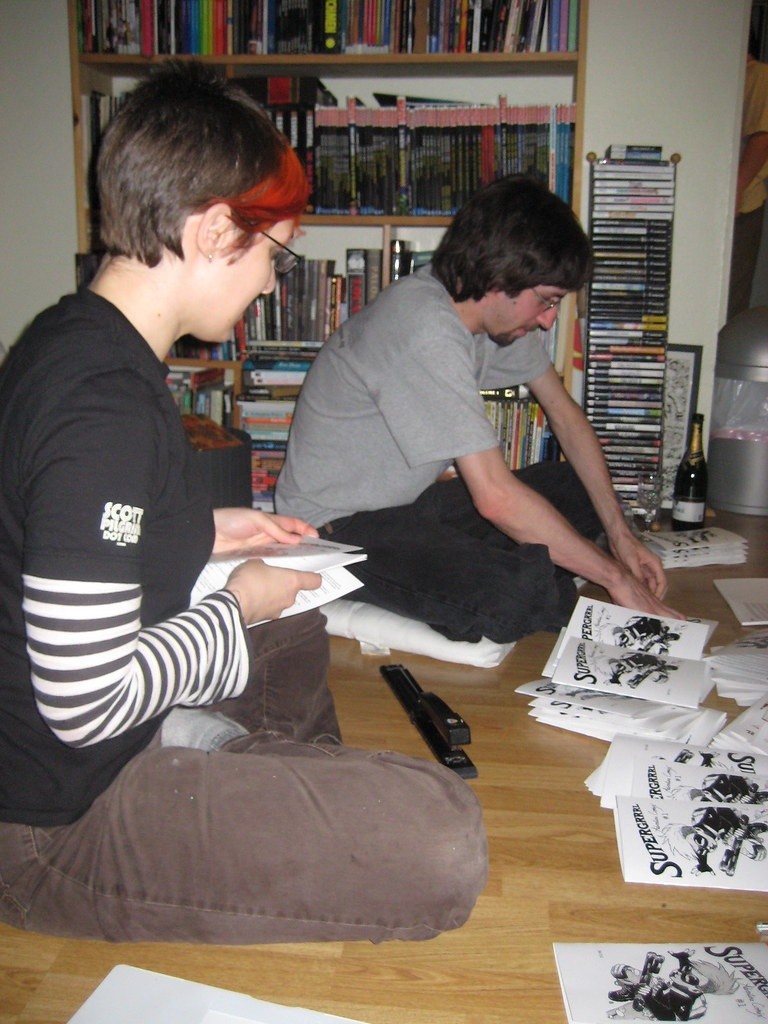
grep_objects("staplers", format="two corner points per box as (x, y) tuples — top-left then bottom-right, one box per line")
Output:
(380, 663), (481, 780)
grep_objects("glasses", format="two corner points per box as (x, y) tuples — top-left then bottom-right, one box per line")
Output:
(244, 216), (301, 274)
(531, 286), (560, 312)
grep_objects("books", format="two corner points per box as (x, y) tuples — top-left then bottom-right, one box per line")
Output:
(167, 239), (435, 513)
(513, 526), (768, 1024)
(481, 320), (557, 472)
(190, 534), (367, 633)
(75, 0), (580, 54)
(584, 145), (674, 518)
(84, 75), (577, 218)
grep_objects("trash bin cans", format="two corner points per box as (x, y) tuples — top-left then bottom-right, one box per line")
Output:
(706, 304), (768, 515)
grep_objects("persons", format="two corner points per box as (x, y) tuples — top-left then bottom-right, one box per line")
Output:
(0, 59), (486, 942)
(728, 27), (768, 321)
(274, 174), (685, 645)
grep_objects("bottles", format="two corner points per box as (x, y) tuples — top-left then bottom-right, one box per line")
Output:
(671, 413), (708, 533)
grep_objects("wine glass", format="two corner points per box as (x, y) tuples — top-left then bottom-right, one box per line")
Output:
(637, 471), (664, 533)
